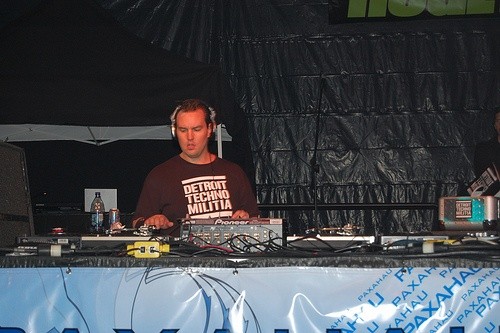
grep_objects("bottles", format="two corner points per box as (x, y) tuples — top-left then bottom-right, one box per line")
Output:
(89, 192), (105, 235)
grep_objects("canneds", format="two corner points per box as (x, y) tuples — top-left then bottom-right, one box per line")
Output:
(108, 208), (120, 227)
(52, 227), (63, 232)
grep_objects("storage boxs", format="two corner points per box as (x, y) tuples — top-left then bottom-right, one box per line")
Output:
(437, 196), (498, 229)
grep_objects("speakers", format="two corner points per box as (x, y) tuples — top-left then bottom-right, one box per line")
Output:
(0, 142), (35, 254)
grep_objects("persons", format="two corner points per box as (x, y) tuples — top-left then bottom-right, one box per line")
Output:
(473, 105), (500, 196)
(129, 99), (260, 237)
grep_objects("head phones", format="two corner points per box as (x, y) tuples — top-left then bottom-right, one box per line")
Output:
(170, 98), (217, 139)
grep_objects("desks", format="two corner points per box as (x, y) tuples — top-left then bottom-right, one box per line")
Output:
(34, 208), (136, 233)
(0, 236), (500, 333)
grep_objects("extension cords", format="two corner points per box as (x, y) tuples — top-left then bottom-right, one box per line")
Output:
(126, 242), (170, 258)
